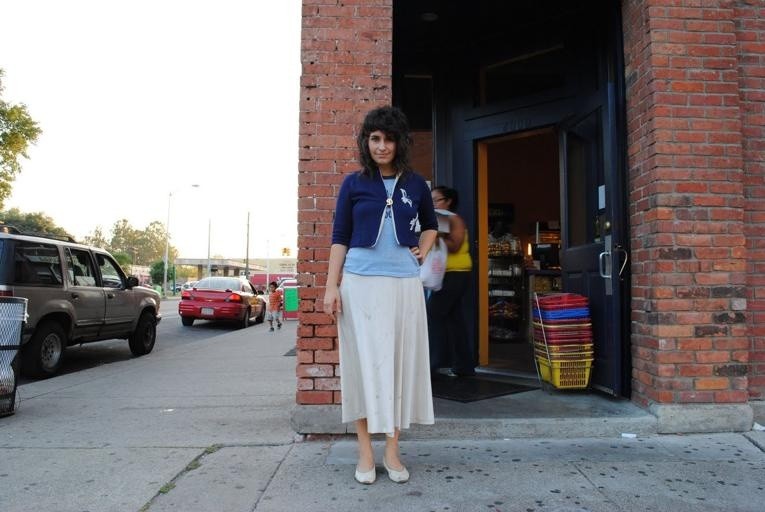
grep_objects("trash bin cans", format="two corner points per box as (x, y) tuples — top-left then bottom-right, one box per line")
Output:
(0, 296), (30, 419)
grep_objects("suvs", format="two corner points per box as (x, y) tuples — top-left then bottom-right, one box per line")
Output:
(1, 225), (161, 378)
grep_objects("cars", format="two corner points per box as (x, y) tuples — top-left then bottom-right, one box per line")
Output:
(176, 277), (297, 326)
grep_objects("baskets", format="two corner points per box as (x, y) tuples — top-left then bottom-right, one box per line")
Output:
(532, 291), (595, 391)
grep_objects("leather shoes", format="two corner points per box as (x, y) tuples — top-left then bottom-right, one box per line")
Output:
(382, 455), (410, 483)
(355, 462), (376, 485)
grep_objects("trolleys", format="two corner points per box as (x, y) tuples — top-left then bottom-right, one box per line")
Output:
(532, 292), (595, 397)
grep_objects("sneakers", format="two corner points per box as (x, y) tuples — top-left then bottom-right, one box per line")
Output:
(269, 322), (282, 330)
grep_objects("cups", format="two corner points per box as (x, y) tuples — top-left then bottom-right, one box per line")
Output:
(513, 264), (521, 275)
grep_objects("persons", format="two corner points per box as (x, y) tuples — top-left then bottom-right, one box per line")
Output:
(426, 185), (473, 380)
(267, 282), (284, 332)
(321, 105), (440, 484)
(275, 276), (281, 287)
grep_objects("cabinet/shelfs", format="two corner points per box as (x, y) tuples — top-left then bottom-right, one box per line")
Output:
(487, 250), (527, 341)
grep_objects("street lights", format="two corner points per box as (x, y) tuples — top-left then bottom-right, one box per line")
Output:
(164, 184), (201, 294)
